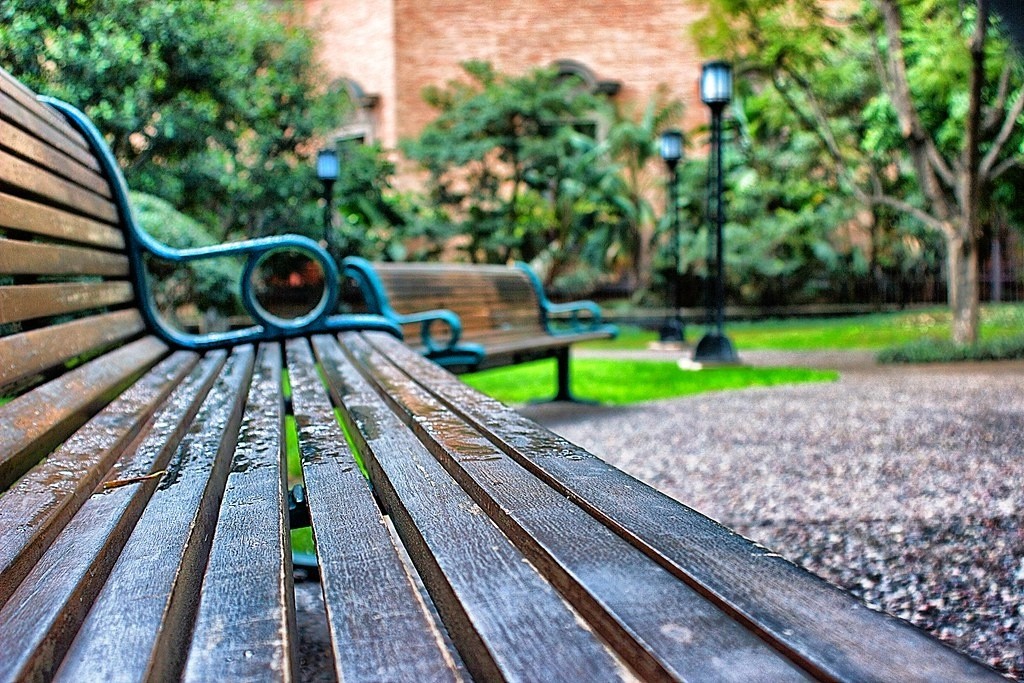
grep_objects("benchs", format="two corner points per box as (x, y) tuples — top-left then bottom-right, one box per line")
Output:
(338, 256), (619, 409)
(0, 65), (1009, 683)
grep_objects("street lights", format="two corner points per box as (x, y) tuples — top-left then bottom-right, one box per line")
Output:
(693, 61), (733, 363)
(661, 129), (685, 342)
(317, 149), (342, 257)
